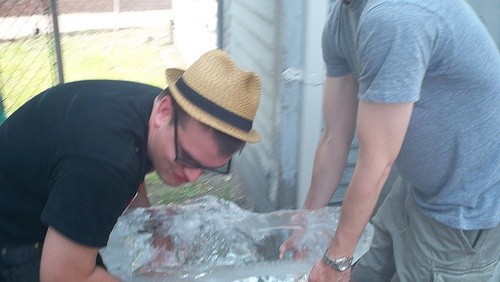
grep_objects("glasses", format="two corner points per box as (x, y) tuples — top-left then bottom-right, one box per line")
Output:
(172, 104), (231, 178)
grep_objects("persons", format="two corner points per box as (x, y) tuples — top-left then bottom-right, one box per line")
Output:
(278, 0), (500, 282)
(0, 48), (264, 281)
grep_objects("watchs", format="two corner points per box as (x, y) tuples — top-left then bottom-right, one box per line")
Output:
(321, 250), (355, 272)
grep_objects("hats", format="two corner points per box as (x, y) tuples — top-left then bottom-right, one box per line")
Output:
(165, 50), (264, 144)
